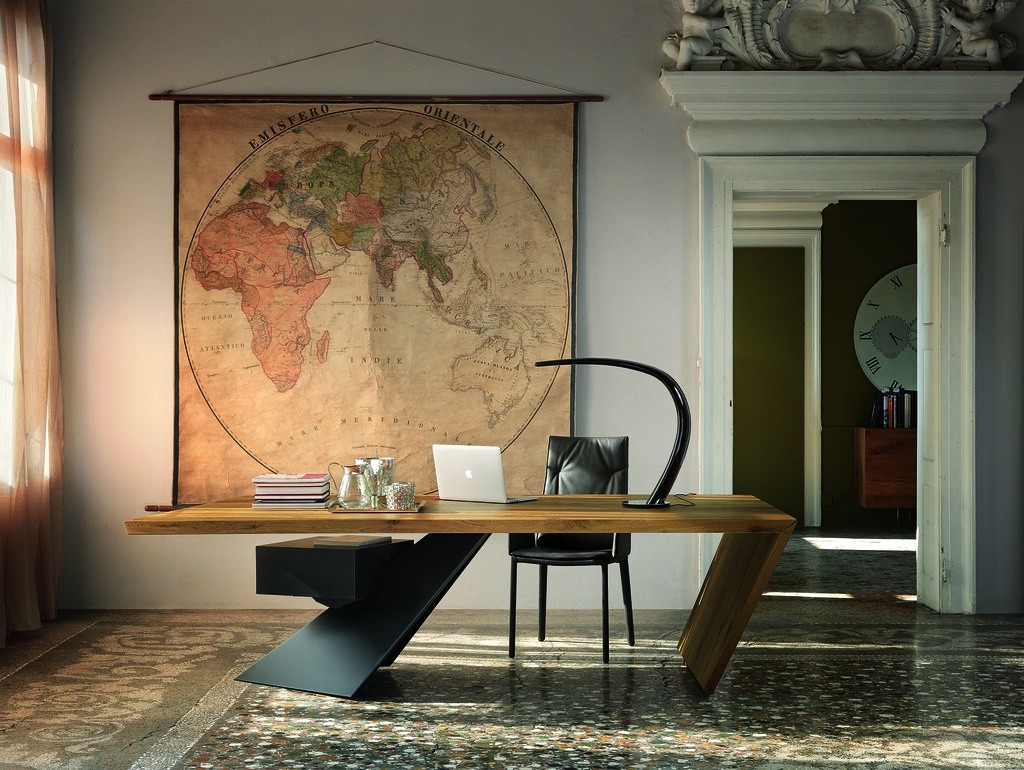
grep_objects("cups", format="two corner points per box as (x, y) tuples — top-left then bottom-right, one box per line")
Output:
(384, 481), (418, 511)
(354, 456), (395, 508)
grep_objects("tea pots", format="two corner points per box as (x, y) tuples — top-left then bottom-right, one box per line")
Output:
(326, 461), (371, 509)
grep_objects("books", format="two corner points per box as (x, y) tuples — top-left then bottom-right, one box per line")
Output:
(251, 473), (331, 509)
(883, 394), (911, 429)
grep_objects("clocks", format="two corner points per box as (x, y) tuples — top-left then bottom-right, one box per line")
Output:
(854, 263), (917, 395)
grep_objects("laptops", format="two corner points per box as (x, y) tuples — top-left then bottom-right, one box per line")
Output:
(432, 444), (539, 503)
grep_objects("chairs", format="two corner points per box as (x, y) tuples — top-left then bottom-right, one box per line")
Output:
(505, 436), (636, 663)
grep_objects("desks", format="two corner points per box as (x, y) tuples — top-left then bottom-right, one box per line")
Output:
(123, 492), (798, 697)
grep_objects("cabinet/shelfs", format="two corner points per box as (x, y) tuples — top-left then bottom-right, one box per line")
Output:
(855, 426), (917, 533)
